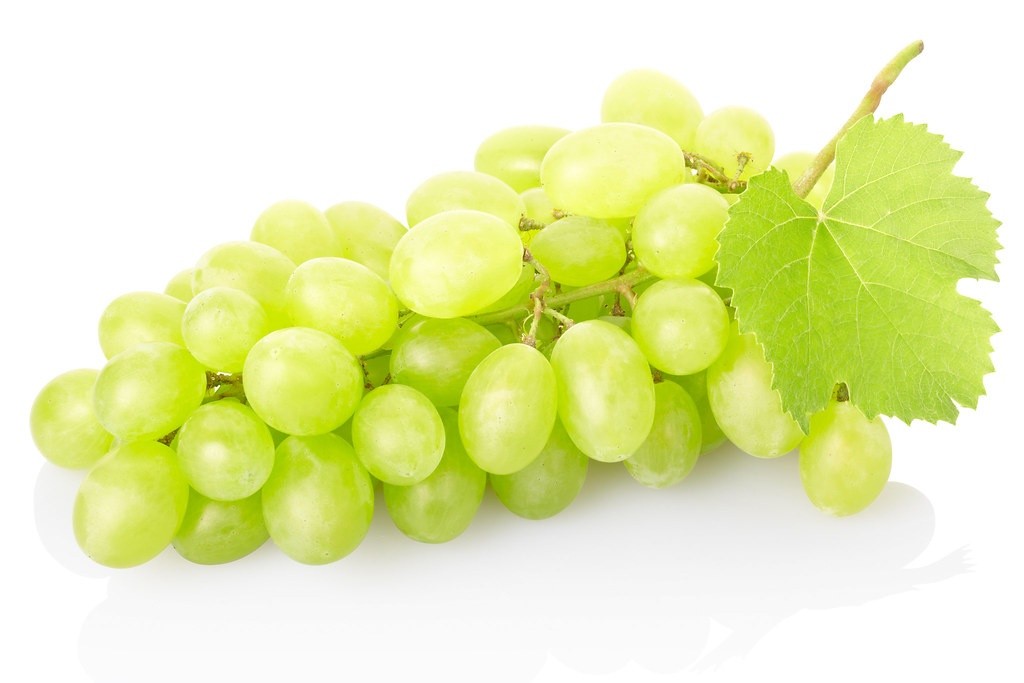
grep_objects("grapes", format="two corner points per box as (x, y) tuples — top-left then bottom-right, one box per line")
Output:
(32, 68), (895, 569)
